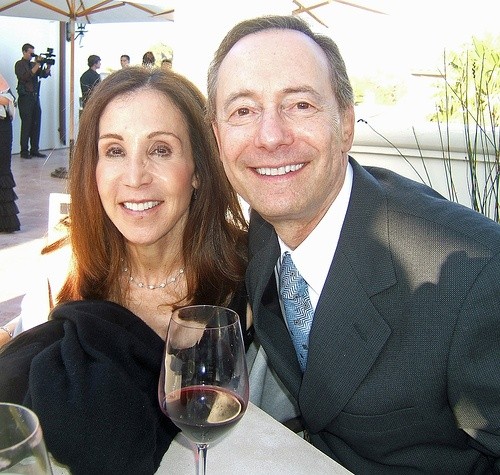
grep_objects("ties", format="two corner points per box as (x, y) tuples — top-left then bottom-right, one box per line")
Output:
(277, 250), (317, 372)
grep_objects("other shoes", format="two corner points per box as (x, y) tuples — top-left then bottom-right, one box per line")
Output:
(29, 151), (46, 158)
(21, 153), (32, 158)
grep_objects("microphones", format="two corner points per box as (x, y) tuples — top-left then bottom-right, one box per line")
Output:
(42, 54), (56, 56)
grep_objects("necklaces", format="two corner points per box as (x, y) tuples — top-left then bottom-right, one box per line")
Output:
(119, 257), (184, 290)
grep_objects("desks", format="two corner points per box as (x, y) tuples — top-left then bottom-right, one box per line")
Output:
(151, 392), (351, 475)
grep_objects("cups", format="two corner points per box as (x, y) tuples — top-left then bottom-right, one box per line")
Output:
(1, 402), (53, 475)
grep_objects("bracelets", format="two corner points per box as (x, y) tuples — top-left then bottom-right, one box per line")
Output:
(0, 326), (14, 339)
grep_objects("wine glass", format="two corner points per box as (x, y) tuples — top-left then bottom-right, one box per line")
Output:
(157, 304), (250, 474)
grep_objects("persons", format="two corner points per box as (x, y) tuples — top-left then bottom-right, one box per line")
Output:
(0, 65), (306, 440)
(143, 51), (156, 66)
(120, 54), (130, 67)
(207, 17), (500, 475)
(0, 72), (21, 233)
(161, 60), (172, 69)
(79, 55), (103, 108)
(15, 43), (53, 158)
